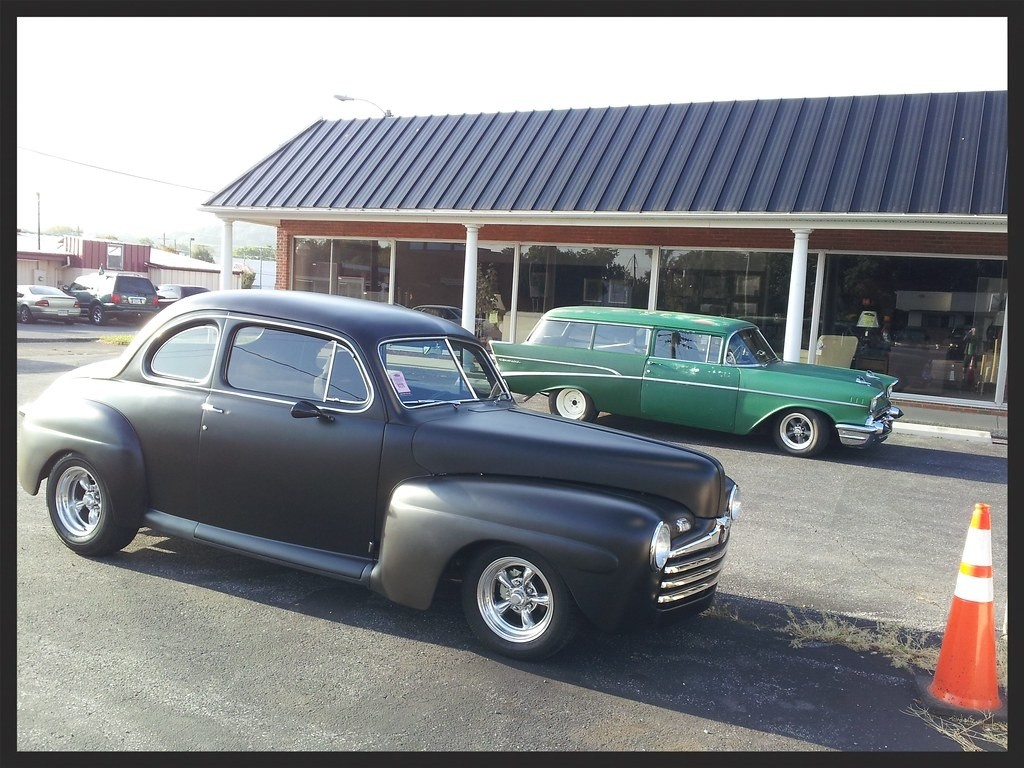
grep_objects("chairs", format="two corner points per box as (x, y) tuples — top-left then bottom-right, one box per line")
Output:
(313, 350), (365, 399)
(657, 333), (703, 362)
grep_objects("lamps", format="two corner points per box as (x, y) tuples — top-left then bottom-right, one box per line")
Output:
(856, 311), (879, 353)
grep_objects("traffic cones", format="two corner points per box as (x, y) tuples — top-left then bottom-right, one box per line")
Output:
(948, 363), (956, 382)
(912, 503), (1007, 726)
(968, 357), (976, 384)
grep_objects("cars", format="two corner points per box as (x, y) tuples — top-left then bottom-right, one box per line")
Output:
(881, 324), (933, 387)
(412, 305), (486, 329)
(17, 285), (82, 325)
(62, 271), (159, 326)
(490, 307), (904, 458)
(153, 284), (209, 311)
(736, 317), (818, 362)
(17, 288), (743, 665)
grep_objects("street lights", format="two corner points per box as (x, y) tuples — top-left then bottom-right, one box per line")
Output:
(190, 238), (195, 257)
